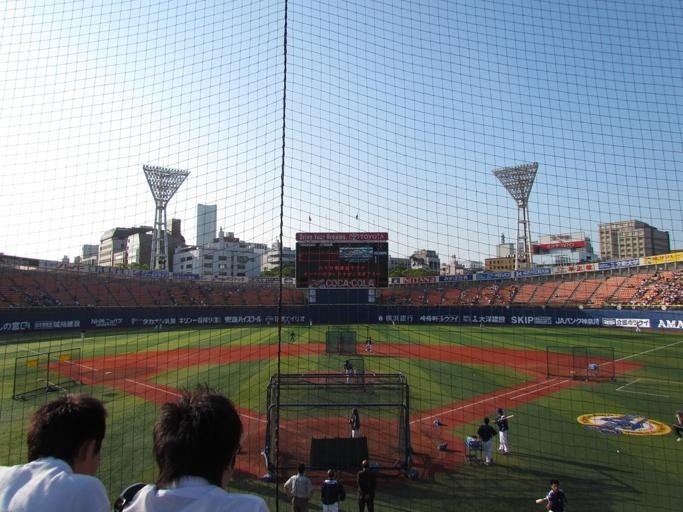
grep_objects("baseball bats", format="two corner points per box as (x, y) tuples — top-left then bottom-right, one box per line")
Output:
(536, 497), (548, 504)
(497, 414), (514, 423)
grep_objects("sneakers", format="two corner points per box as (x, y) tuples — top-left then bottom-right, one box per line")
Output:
(677, 437), (683, 442)
(482, 458), (493, 465)
(498, 448), (512, 455)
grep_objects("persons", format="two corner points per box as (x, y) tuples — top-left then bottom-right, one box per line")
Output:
(289, 330), (294, 343)
(318, 468), (342, 512)
(347, 407), (360, 438)
(477, 416), (498, 466)
(343, 359), (355, 383)
(672, 412), (682, 442)
(116, 381), (270, 512)
(492, 408), (511, 456)
(283, 462), (314, 511)
(363, 336), (373, 352)
(356, 459), (375, 511)
(0, 393), (113, 512)
(374, 284), (520, 307)
(537, 479), (567, 512)
(617, 266), (682, 310)
